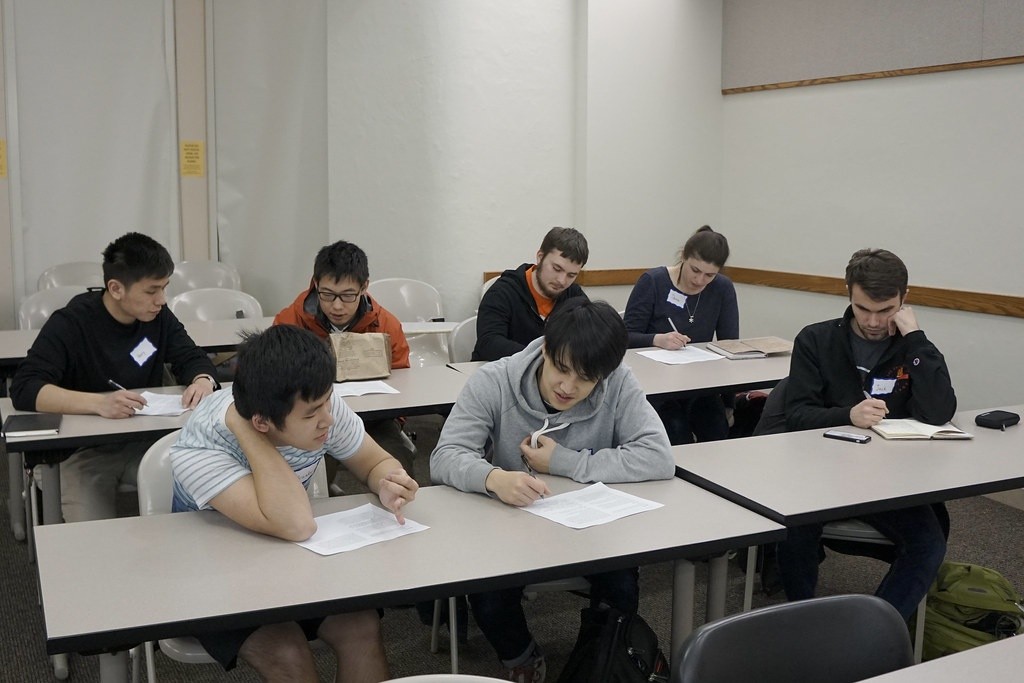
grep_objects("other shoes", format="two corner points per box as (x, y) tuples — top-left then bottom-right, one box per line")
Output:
(509, 650), (546, 683)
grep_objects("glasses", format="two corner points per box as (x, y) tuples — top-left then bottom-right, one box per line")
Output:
(317, 279), (361, 303)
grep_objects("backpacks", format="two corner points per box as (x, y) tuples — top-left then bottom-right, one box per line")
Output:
(908, 557), (1024, 660)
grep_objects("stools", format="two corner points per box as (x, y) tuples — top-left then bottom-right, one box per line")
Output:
(429, 574), (592, 683)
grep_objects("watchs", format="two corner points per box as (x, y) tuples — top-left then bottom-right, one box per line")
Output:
(194, 375), (215, 383)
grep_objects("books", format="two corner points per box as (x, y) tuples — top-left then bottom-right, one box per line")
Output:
(869, 419), (974, 440)
(0, 412), (63, 438)
(706, 336), (793, 360)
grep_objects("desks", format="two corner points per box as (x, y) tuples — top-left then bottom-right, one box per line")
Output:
(0, 320), (1024, 683)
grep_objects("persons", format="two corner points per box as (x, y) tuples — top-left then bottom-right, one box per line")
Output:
(430, 297), (675, 683)
(171, 323), (419, 683)
(624, 224), (740, 445)
(273, 240), (413, 490)
(9, 232), (221, 523)
(469, 227), (589, 362)
(756, 249), (958, 618)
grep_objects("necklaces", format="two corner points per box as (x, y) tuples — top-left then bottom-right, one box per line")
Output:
(684, 292), (701, 323)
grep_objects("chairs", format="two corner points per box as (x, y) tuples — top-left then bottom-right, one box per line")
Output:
(743, 372), (897, 611)
(680, 594), (918, 683)
(20, 258), (503, 455)
(131, 427), (341, 682)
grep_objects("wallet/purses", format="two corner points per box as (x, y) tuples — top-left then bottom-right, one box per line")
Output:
(975, 409), (1021, 431)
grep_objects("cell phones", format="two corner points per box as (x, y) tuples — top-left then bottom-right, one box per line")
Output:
(823, 430), (872, 444)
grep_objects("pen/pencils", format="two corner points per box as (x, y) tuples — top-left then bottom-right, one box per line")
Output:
(668, 317), (687, 350)
(863, 390), (887, 419)
(521, 454), (544, 500)
(108, 380), (150, 408)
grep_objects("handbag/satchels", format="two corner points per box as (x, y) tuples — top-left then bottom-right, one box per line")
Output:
(563, 606), (668, 683)
